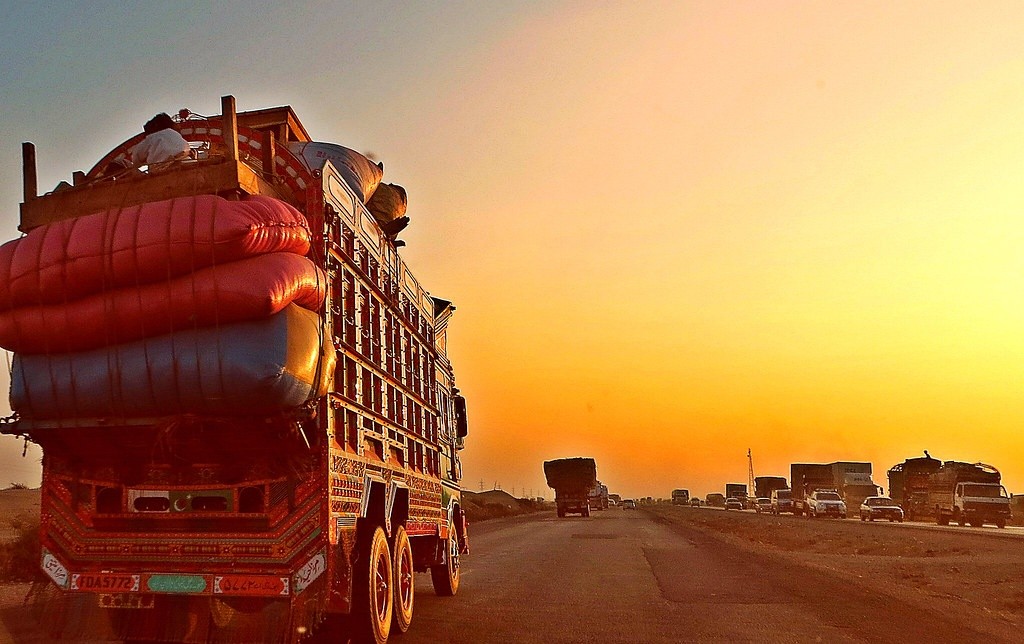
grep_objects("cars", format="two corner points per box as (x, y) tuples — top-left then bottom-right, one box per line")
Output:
(608, 499), (616, 506)
(617, 500), (623, 506)
(859, 496), (904, 523)
(754, 497), (773, 514)
(623, 499), (635, 510)
(724, 497), (742, 510)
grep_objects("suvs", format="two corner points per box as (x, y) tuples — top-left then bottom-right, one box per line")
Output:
(803, 490), (848, 520)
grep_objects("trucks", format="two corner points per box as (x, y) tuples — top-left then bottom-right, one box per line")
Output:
(554, 480), (620, 517)
(792, 461), (884, 518)
(886, 462), (1012, 530)
(671, 472), (794, 515)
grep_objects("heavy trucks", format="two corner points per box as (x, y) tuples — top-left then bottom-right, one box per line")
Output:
(0, 119), (474, 644)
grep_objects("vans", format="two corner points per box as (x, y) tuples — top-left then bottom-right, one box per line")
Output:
(690, 497), (700, 507)
